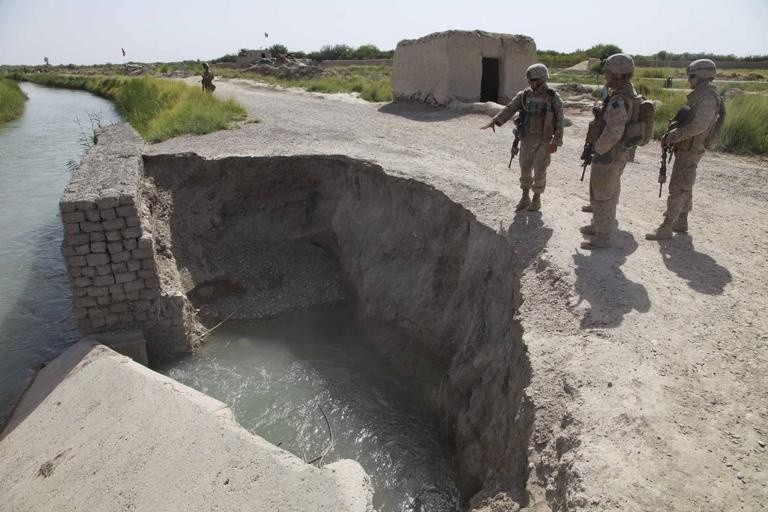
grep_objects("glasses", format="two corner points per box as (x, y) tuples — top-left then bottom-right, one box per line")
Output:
(530, 79), (538, 82)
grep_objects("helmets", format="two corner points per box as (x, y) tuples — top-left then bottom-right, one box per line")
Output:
(601, 52), (634, 77)
(686, 58), (717, 79)
(526, 63), (550, 81)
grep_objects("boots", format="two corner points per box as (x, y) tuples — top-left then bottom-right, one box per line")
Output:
(527, 191), (542, 212)
(645, 217), (675, 241)
(673, 211), (690, 232)
(513, 189), (532, 210)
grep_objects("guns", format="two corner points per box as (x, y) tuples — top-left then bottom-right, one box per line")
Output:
(508, 111), (524, 168)
(659, 134), (669, 197)
(581, 107), (603, 181)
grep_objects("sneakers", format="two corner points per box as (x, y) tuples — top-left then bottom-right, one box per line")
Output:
(579, 203), (612, 250)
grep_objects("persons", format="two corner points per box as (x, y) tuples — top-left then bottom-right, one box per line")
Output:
(480, 63), (563, 211)
(644, 59), (726, 239)
(580, 83), (654, 222)
(201, 62), (215, 93)
(578, 54), (639, 249)
